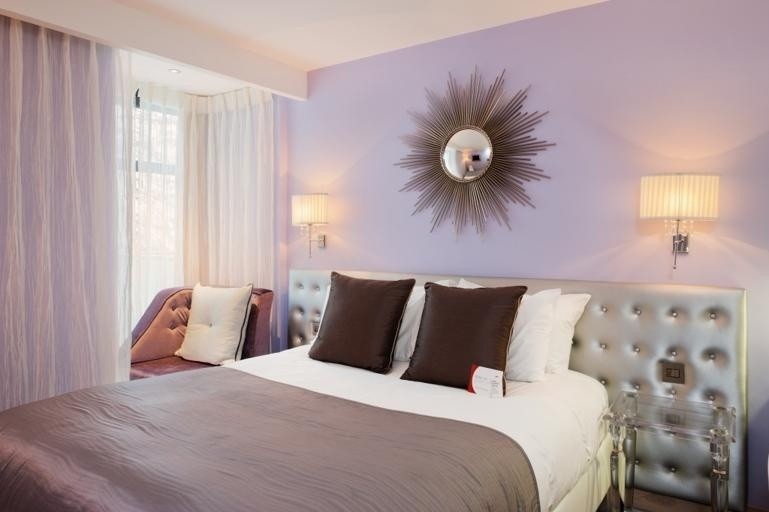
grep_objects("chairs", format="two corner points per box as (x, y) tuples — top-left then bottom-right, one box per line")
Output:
(119, 286), (274, 379)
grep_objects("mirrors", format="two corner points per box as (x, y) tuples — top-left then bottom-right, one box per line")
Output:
(436, 124), (495, 186)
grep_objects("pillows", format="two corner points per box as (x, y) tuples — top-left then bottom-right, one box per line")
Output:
(173, 282), (253, 367)
(306, 272), (589, 395)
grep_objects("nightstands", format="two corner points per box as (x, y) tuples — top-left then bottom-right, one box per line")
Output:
(602, 390), (738, 509)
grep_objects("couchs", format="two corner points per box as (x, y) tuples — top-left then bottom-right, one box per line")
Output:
(2, 343), (609, 510)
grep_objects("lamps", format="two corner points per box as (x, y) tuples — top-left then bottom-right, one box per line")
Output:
(636, 175), (722, 268)
(289, 193), (327, 259)
